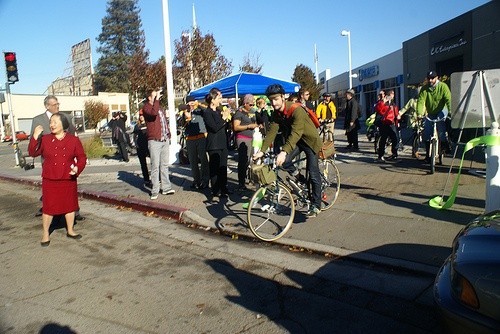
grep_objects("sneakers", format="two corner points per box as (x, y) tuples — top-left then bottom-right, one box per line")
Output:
(412, 150), (419, 158)
(377, 155), (386, 161)
(151, 192), (158, 200)
(388, 152), (398, 160)
(162, 189), (175, 195)
(144, 182), (153, 191)
(305, 205), (321, 219)
(190, 184), (209, 191)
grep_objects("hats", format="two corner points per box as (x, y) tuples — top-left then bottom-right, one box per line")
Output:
(183, 95), (198, 104)
(427, 71), (437, 78)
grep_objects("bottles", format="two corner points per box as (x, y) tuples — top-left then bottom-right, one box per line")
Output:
(286, 176), (302, 193)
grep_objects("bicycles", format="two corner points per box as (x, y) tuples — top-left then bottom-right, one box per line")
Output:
(413, 115), (450, 170)
(366, 121), (403, 158)
(247, 144), (336, 240)
(317, 119), (335, 144)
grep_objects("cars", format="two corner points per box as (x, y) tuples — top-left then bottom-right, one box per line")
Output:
(2, 130), (28, 142)
(431, 209), (500, 334)
(98, 120), (136, 132)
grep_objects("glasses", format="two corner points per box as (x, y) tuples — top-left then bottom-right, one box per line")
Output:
(249, 104), (255, 107)
(50, 103), (59, 106)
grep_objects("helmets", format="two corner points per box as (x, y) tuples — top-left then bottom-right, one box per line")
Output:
(265, 84), (286, 96)
(139, 108), (145, 114)
(321, 92), (332, 97)
(346, 89), (355, 95)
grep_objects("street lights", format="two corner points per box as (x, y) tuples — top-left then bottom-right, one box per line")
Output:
(341, 29), (359, 91)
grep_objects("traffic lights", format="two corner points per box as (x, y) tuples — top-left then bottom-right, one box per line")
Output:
(6, 52), (18, 82)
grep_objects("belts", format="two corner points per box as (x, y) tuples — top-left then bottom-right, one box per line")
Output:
(185, 132), (207, 141)
(154, 140), (167, 142)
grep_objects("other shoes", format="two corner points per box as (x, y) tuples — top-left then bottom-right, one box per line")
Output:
(73, 214), (85, 221)
(41, 240), (50, 247)
(67, 232), (82, 239)
(345, 145), (360, 153)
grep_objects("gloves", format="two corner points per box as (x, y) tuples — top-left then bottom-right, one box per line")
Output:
(444, 113), (452, 126)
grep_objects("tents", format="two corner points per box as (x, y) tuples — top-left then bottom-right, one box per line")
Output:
(190, 70), (301, 110)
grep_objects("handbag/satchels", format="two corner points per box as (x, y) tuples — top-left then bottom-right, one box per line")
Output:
(123, 133), (130, 144)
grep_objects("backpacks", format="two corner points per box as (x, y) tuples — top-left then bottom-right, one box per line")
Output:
(287, 103), (320, 129)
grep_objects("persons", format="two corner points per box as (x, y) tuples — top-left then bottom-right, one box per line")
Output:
(397, 87), (428, 157)
(316, 92), (336, 156)
(202, 89), (232, 203)
(219, 94), (274, 189)
(133, 109), (161, 184)
(28, 113), (87, 245)
(375, 90), (399, 162)
(108, 112), (129, 162)
(142, 90), (175, 199)
(30, 95), (84, 220)
(252, 84), (321, 217)
(289, 88), (312, 167)
(416, 71), (450, 161)
(345, 88), (359, 152)
(176, 96), (209, 189)
